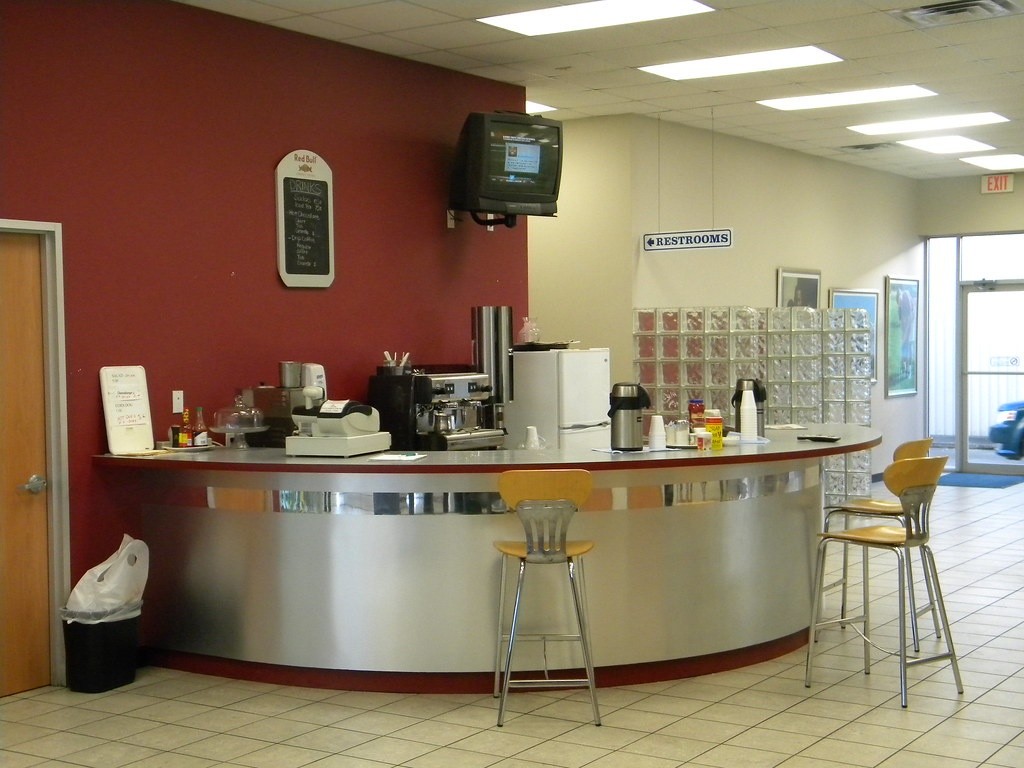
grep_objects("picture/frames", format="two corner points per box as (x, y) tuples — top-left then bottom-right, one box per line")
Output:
(885, 275), (919, 398)
(828, 289), (878, 384)
(777, 267), (822, 308)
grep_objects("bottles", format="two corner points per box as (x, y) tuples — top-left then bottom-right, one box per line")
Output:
(667, 416), (714, 452)
(193, 407), (208, 447)
(179, 408), (193, 449)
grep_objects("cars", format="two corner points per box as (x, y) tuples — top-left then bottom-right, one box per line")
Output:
(987, 401), (1024, 459)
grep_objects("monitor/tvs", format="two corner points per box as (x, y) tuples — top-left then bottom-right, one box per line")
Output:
(453, 112), (564, 204)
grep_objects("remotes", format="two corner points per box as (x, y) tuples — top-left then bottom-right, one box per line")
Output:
(797, 434), (840, 442)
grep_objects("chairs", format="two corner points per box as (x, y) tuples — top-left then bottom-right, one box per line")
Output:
(493, 468), (603, 727)
(804, 438), (965, 708)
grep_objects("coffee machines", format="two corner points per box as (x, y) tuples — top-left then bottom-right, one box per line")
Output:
(367, 359), (507, 451)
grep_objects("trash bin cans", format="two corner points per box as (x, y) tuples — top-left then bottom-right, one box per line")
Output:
(59, 600), (144, 694)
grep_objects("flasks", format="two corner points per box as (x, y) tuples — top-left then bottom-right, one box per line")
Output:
(731, 378), (765, 438)
(608, 382), (646, 450)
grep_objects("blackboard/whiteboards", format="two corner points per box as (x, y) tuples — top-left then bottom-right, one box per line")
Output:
(275, 150), (335, 289)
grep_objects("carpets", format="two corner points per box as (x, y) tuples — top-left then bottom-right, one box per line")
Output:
(936, 472), (1024, 488)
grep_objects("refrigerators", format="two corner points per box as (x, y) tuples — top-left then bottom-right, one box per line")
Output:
(504, 346), (612, 451)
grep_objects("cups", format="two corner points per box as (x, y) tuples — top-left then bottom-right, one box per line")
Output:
(524, 426), (540, 450)
(740, 391), (758, 440)
(648, 415), (667, 451)
